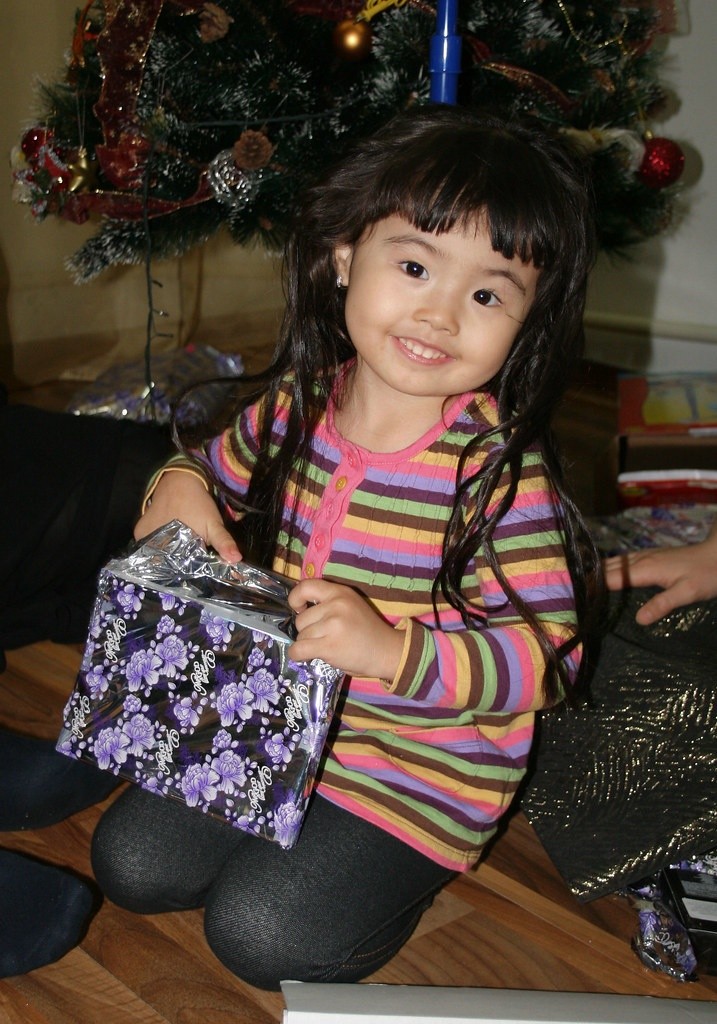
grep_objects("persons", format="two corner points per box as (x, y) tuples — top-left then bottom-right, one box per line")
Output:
(584, 518), (717, 625)
(89, 114), (598, 994)
(0, 644), (129, 983)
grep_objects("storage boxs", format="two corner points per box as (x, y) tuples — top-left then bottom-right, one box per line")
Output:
(608, 378), (717, 509)
(54, 561), (347, 850)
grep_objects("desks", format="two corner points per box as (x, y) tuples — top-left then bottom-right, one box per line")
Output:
(0, 640), (717, 1024)
